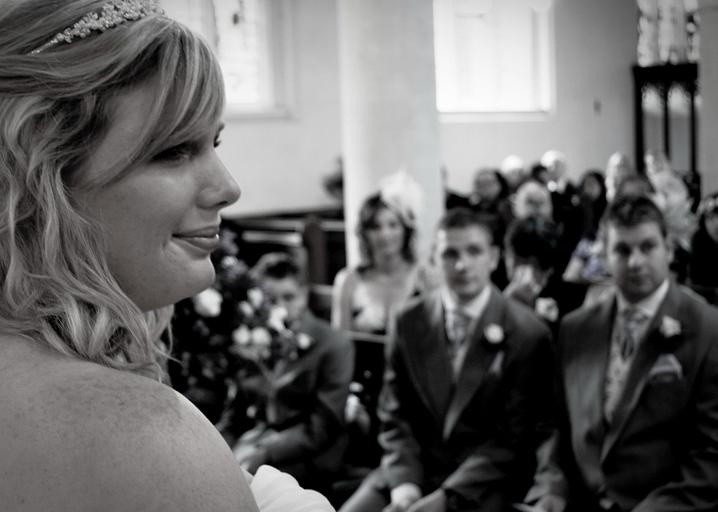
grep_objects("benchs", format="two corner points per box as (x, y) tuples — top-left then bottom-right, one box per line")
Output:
(233, 217), (386, 477)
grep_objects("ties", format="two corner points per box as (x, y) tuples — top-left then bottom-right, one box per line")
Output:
(620, 309), (648, 359)
(448, 311), (474, 360)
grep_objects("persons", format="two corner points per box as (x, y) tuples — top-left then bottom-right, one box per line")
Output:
(322, 172), (431, 341)
(331, 210), (560, 511)
(441, 150), (718, 328)
(199, 247), (358, 498)
(516, 193), (718, 510)
(0, 0), (265, 511)
